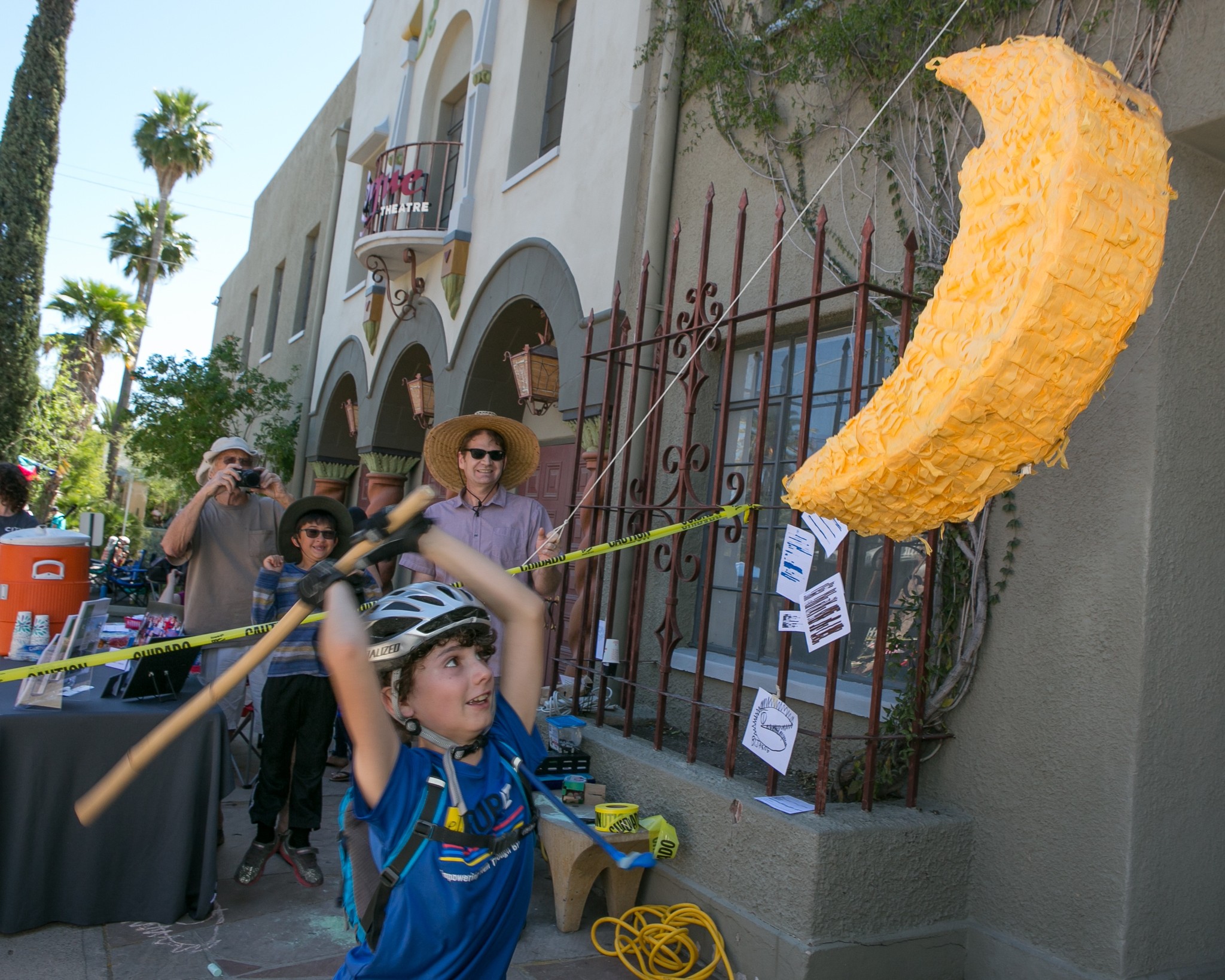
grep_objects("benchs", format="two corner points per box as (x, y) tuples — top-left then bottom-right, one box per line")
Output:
(531, 790), (649, 934)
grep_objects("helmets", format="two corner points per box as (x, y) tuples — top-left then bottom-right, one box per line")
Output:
(358, 582), (496, 671)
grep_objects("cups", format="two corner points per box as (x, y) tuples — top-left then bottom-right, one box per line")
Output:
(602, 639), (620, 676)
(9, 611), (32, 660)
(29, 615), (50, 661)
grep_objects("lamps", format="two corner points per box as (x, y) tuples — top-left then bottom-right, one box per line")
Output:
(501, 301), (560, 418)
(401, 363), (436, 429)
(340, 394), (359, 439)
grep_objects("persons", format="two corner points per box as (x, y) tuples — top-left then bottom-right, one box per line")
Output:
(395, 411), (562, 677)
(0, 462), (38, 535)
(233, 495), (382, 886)
(312, 508), (551, 980)
(49, 506), (67, 532)
(159, 436), (295, 854)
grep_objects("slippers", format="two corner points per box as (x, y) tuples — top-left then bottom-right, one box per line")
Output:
(329, 771), (352, 782)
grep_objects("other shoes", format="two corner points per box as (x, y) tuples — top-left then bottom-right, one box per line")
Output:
(279, 833), (324, 888)
(235, 832), (281, 885)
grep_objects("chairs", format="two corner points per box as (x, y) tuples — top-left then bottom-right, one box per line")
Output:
(88, 535), (169, 608)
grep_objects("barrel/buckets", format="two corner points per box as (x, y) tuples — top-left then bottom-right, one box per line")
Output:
(0, 524), (91, 655)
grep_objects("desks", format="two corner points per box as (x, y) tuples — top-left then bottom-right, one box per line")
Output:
(0, 652), (232, 935)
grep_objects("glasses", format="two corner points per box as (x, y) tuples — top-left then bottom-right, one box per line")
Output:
(461, 448), (506, 462)
(223, 458), (252, 469)
(298, 527), (339, 539)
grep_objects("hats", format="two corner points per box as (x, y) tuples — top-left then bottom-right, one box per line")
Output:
(52, 506), (59, 510)
(277, 496), (354, 562)
(423, 411), (541, 494)
(195, 437), (261, 487)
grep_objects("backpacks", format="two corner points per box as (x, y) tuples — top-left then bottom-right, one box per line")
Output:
(337, 746), (541, 951)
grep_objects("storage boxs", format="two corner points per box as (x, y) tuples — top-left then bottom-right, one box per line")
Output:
(562, 774), (588, 806)
(546, 713), (587, 754)
(537, 746), (591, 775)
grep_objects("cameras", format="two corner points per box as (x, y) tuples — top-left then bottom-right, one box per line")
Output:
(232, 469), (262, 488)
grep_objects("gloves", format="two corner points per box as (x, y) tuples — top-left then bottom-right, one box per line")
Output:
(346, 504), (435, 570)
(296, 561), (350, 610)
